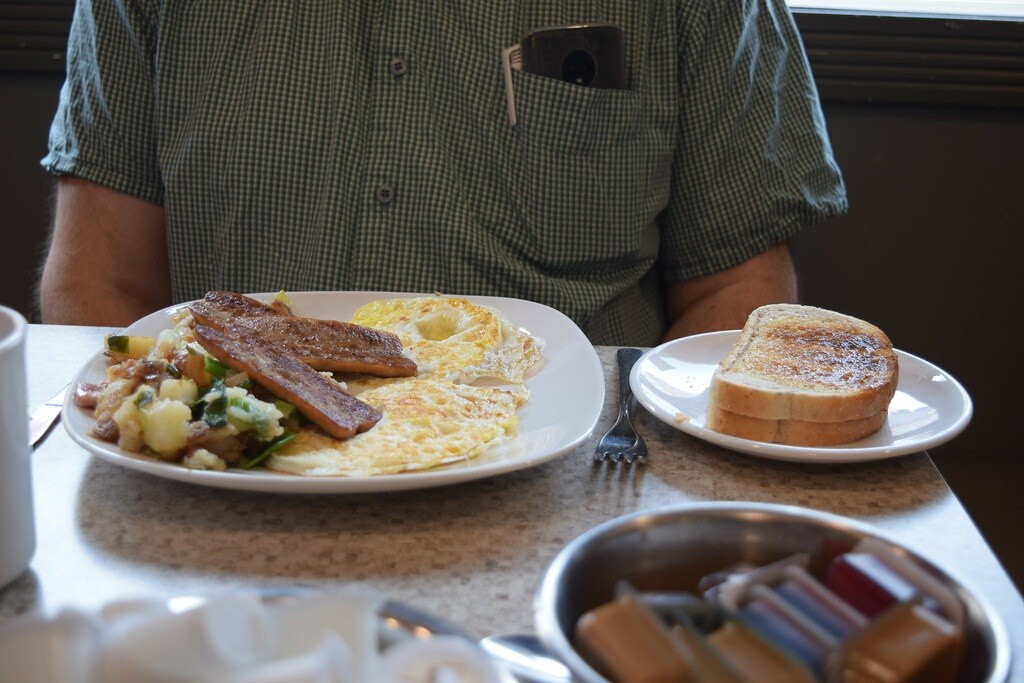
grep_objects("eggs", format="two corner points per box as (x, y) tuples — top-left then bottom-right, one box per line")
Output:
(267, 376), (520, 478)
(331, 296), (548, 386)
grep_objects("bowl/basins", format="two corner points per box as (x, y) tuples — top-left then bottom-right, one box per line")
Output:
(535, 499), (1013, 683)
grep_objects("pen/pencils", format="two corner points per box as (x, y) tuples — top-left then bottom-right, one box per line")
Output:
(501, 44), (523, 128)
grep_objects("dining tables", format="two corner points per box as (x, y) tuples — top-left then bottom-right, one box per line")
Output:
(0, 324), (1024, 683)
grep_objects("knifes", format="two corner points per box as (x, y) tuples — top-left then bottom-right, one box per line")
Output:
(28, 381), (72, 445)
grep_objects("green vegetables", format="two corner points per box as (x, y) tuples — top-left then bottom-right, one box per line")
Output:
(188, 357), (299, 468)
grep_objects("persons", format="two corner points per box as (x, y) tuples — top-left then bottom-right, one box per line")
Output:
(40, 0), (849, 344)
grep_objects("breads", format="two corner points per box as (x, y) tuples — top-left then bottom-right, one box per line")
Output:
(707, 303), (899, 447)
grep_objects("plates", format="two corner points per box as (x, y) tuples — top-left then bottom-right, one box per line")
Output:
(61, 289), (607, 494)
(628, 328), (974, 464)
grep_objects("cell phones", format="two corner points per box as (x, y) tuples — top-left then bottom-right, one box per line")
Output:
(522, 23), (624, 92)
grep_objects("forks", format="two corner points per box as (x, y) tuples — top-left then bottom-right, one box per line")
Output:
(594, 348), (650, 468)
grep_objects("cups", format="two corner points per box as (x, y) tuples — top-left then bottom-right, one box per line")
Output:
(0, 303), (37, 590)
